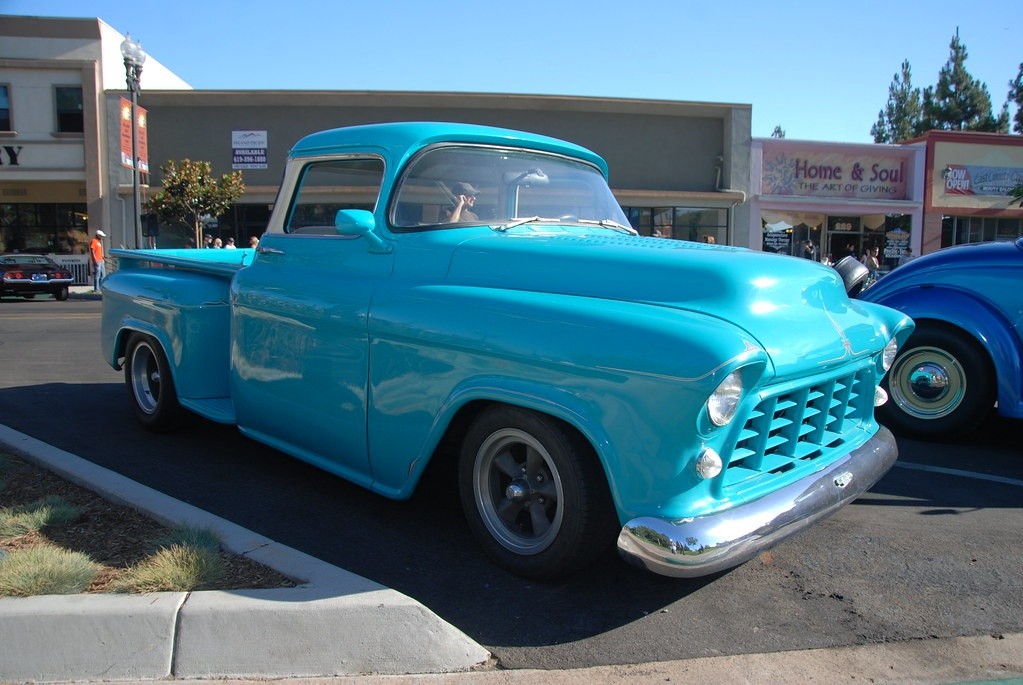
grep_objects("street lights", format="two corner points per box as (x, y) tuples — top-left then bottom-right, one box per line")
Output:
(120, 33), (147, 249)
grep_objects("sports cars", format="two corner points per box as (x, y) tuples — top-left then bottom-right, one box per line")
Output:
(0, 254), (75, 301)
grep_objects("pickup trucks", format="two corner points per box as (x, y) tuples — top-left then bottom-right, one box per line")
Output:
(97, 121), (916, 580)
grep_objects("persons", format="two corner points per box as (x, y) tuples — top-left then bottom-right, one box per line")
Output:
(90, 230), (107, 291)
(703, 232), (916, 281)
(203, 234), (259, 249)
(438, 182), (479, 221)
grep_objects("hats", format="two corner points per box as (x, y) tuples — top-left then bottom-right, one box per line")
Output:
(452, 182), (480, 195)
(228, 237), (235, 242)
(903, 248), (912, 252)
(205, 234), (213, 239)
(96, 230), (106, 236)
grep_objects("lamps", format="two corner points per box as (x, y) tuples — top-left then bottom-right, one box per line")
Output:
(942, 165), (952, 179)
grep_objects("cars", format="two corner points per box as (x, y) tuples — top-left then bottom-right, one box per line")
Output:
(835, 233), (1023, 449)
